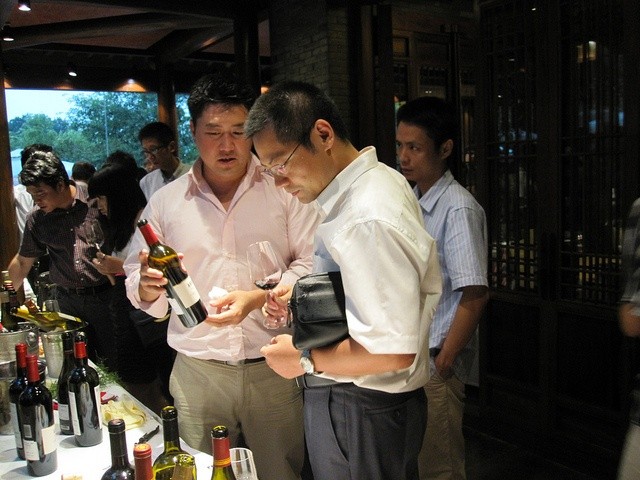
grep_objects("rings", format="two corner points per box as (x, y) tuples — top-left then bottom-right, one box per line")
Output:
(96, 262), (101, 267)
(100, 254), (106, 261)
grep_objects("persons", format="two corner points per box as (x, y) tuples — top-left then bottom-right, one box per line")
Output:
(396, 96), (491, 480)
(88, 164), (171, 408)
(72, 150), (154, 200)
(14, 144), (53, 306)
(618, 197), (640, 480)
(243, 80), (443, 480)
(138, 122), (192, 202)
(122, 73), (320, 480)
(7, 151), (113, 373)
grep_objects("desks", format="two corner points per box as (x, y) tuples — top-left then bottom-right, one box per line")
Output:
(1, 359), (189, 479)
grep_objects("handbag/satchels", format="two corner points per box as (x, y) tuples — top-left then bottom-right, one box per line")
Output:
(128, 304), (171, 350)
(287, 272), (348, 350)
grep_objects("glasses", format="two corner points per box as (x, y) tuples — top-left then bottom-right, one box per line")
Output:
(260, 143), (300, 179)
(144, 146), (166, 156)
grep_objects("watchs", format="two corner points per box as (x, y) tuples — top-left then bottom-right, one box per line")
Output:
(300, 348), (323, 376)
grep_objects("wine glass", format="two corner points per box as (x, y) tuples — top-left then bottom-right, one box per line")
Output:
(80, 219), (105, 252)
(229, 447), (258, 480)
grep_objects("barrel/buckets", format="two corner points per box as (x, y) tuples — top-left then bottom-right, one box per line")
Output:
(0, 322), (39, 381)
(38, 322), (89, 378)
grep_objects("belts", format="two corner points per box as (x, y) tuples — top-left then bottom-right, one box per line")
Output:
(227, 357), (265, 367)
(56, 281), (112, 295)
(430, 348), (441, 356)
(296, 374), (339, 390)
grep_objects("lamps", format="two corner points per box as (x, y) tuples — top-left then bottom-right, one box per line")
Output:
(17, 0), (32, 13)
(69, 55), (80, 78)
(2, 25), (15, 43)
(127, 61), (137, 80)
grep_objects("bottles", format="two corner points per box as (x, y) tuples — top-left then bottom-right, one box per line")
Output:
(100, 419), (134, 480)
(246, 241), (287, 330)
(133, 443), (155, 480)
(58, 332), (75, 436)
(137, 218), (208, 329)
(210, 424), (236, 480)
(153, 405), (197, 480)
(67, 335), (103, 447)
(8, 343), (29, 459)
(170, 453), (193, 480)
(10, 306), (88, 333)
(0, 271), (20, 333)
(39, 284), (64, 378)
(19, 355), (57, 477)
